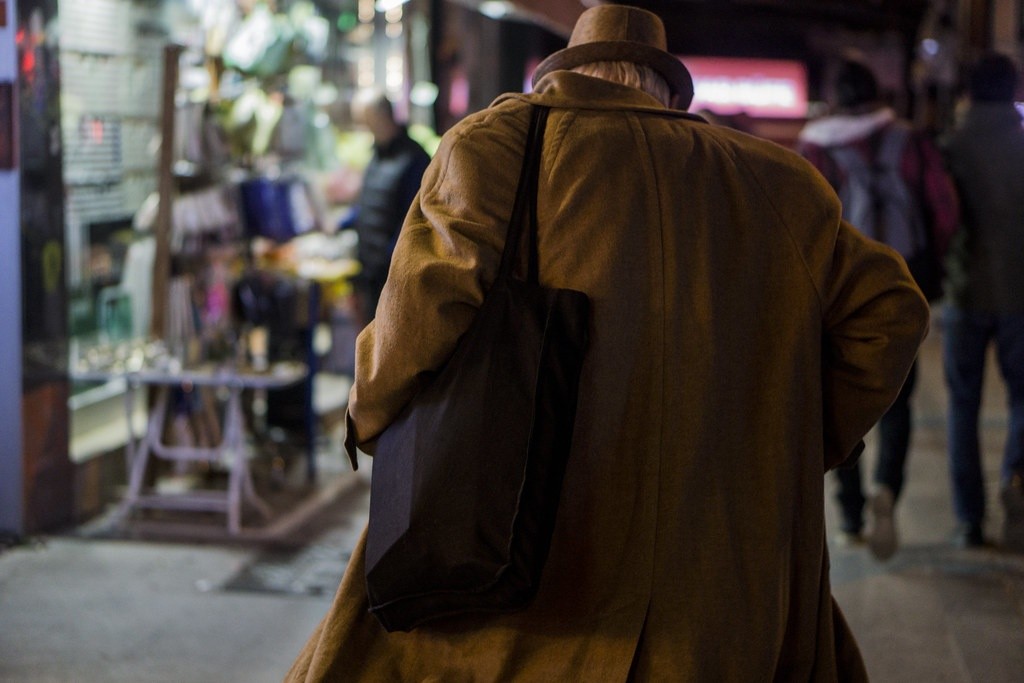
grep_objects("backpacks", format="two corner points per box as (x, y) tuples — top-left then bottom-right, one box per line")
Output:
(825, 118), (928, 278)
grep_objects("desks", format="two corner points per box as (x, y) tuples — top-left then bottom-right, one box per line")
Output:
(67, 346), (314, 531)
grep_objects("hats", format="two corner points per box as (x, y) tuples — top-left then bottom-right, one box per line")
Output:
(531, 5), (694, 111)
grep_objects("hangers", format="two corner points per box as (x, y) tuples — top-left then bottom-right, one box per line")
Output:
(170, 161), (223, 196)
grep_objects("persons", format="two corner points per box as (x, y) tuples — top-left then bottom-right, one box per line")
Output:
(799, 55), (1024, 564)
(282, 3), (932, 682)
(326, 96), (432, 329)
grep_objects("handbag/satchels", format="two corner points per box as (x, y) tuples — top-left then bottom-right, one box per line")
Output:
(363, 101), (596, 633)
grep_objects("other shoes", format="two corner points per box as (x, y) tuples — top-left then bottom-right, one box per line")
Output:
(870, 494), (896, 556)
(965, 522), (983, 545)
(843, 529), (868, 546)
(1001, 486), (1024, 548)
